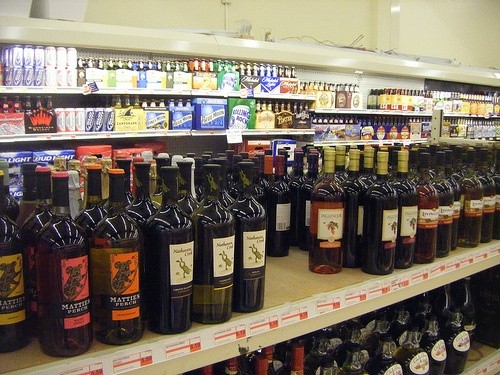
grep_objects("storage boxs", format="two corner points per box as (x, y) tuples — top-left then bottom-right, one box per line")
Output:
(310, 124), (361, 142)
(0, 99), (296, 199)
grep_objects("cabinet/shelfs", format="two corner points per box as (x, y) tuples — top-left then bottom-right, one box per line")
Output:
(309, 108), (500, 148)
(0, 16), (316, 147)
(0, 227), (500, 375)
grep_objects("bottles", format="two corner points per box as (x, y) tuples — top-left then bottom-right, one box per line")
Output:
(0, 57), (500, 375)
